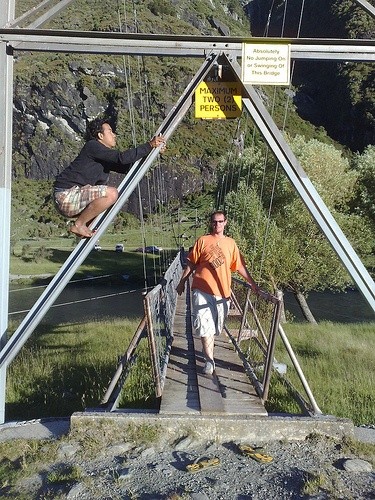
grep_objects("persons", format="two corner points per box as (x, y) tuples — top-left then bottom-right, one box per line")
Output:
(175, 211), (259, 374)
(53, 121), (166, 236)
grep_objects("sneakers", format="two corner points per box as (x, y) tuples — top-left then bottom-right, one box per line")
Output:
(202, 358), (216, 375)
(201, 349), (208, 363)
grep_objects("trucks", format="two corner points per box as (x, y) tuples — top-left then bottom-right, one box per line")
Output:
(116, 244), (124, 253)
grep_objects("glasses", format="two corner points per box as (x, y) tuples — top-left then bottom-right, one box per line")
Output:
(212, 220), (223, 223)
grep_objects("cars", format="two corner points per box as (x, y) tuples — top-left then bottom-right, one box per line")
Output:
(139, 246), (159, 253)
(95, 245), (101, 250)
